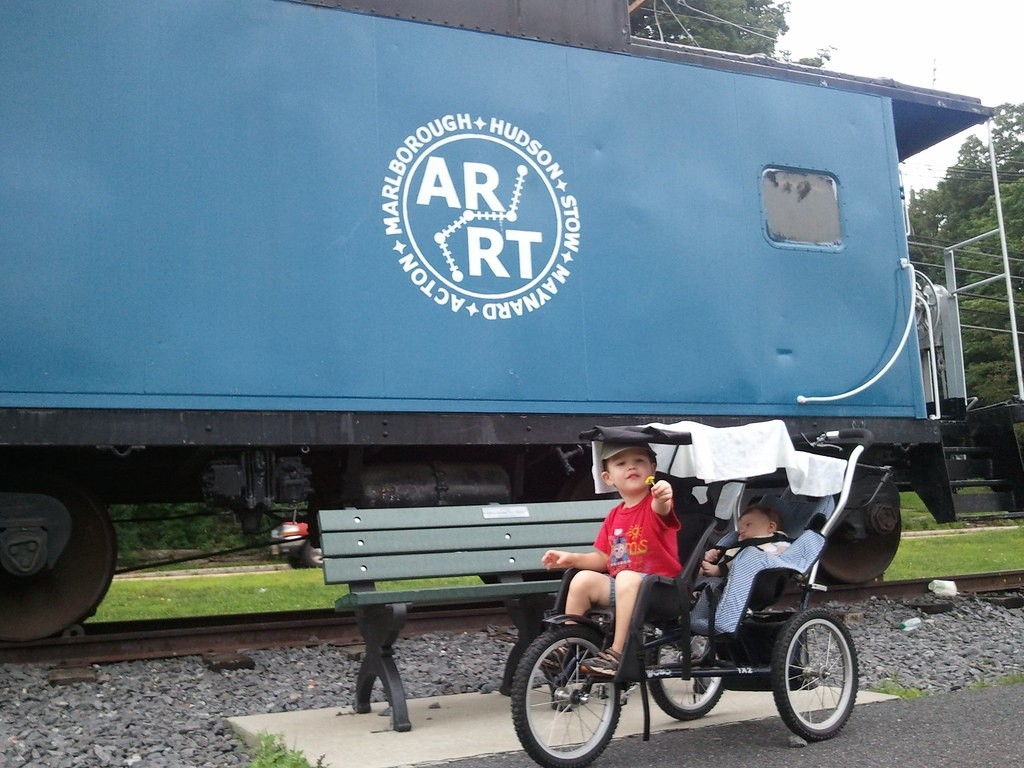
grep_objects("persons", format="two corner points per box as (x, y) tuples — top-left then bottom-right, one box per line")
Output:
(541, 440), (683, 679)
(698, 504), (781, 576)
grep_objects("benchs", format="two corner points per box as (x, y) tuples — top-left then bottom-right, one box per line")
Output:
(315, 499), (625, 732)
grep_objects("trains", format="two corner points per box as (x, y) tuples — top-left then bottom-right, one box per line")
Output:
(0, 0), (1018, 651)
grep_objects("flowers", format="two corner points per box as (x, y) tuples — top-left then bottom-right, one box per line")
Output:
(645, 476), (655, 487)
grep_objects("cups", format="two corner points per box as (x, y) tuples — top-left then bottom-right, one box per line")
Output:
(928, 580), (957, 596)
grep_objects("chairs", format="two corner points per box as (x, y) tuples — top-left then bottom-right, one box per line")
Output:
(678, 485), (838, 636)
(543, 509), (717, 685)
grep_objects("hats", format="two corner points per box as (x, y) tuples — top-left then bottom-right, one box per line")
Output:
(600, 441), (658, 458)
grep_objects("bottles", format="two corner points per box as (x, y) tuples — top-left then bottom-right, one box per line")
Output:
(899, 617), (921, 631)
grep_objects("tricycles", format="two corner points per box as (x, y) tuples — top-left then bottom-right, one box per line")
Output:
(509, 421), (904, 768)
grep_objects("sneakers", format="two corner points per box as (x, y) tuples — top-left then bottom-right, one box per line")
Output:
(580, 647), (622, 679)
(543, 648), (568, 668)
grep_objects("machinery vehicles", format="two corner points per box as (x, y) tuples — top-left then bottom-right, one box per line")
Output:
(269, 512), (326, 569)
